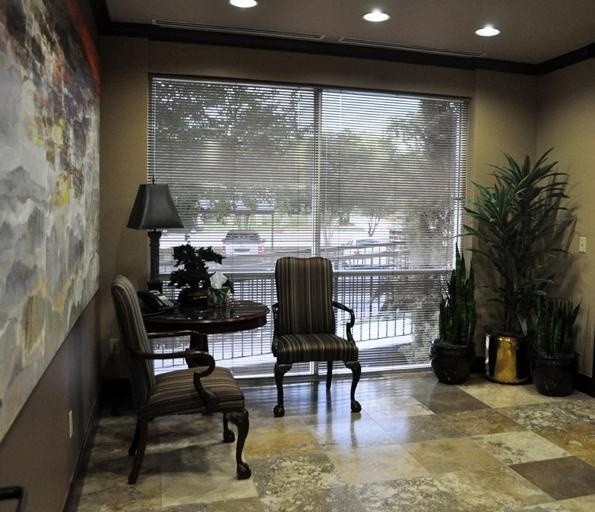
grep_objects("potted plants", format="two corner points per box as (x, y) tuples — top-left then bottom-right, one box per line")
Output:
(457, 146), (578, 386)
(167, 243), (224, 316)
(535, 295), (583, 397)
(428, 242), (478, 383)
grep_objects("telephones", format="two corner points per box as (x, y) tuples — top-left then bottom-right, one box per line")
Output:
(138, 289), (176, 313)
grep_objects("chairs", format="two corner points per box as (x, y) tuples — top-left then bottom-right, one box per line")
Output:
(272, 256), (362, 416)
(112, 273), (253, 485)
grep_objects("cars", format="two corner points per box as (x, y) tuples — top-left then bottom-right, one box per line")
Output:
(222, 230), (267, 257)
(342, 239), (386, 270)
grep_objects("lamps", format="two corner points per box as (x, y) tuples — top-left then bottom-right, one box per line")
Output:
(125, 182), (184, 292)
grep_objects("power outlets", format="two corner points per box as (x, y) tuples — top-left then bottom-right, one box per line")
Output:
(578, 237), (588, 257)
(67, 409), (77, 439)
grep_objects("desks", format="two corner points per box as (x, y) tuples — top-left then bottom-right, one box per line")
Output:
(123, 300), (270, 368)
